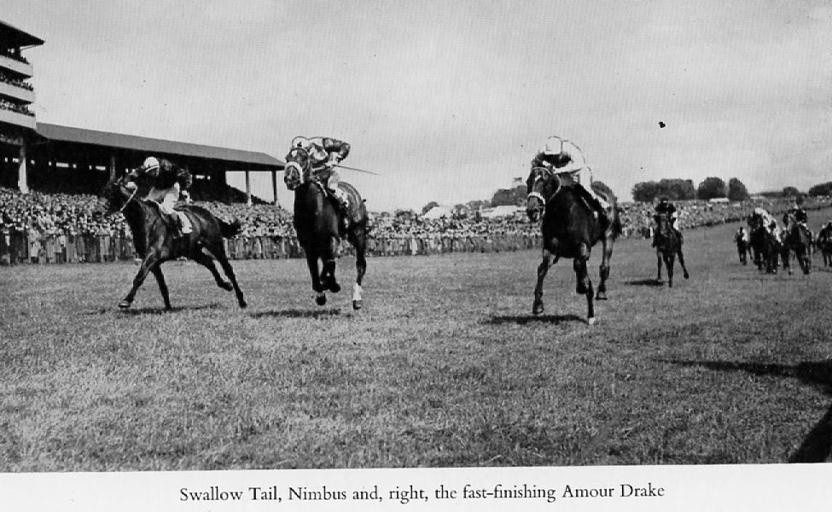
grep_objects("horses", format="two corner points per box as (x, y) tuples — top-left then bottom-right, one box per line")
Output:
(525, 155), (623, 325)
(283, 143), (368, 311)
(736, 206), (832, 275)
(655, 210), (689, 288)
(103, 175), (247, 314)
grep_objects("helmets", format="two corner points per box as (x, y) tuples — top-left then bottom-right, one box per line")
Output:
(543, 136), (564, 156)
(292, 134), (311, 151)
(142, 156), (160, 175)
